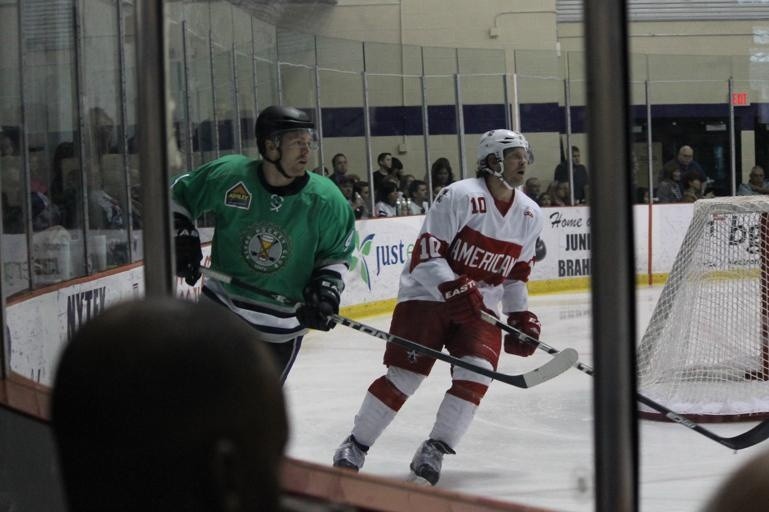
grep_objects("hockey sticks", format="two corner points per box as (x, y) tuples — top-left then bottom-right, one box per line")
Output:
(480, 310), (768, 450)
(196, 266), (578, 388)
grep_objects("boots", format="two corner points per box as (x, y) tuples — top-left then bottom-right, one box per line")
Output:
(333, 435), (369, 475)
(410, 439), (457, 485)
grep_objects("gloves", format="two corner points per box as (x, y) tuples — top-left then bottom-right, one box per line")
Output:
(295, 274), (346, 332)
(172, 212), (201, 285)
(439, 273), (486, 330)
(253, 105), (320, 145)
(478, 128), (531, 162)
(503, 311), (543, 358)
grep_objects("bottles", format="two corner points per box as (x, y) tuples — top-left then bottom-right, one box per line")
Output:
(354, 192), (362, 200)
(396, 198), (411, 216)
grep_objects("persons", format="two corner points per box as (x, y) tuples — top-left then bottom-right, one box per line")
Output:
(170, 105), (357, 387)
(656, 146), (715, 202)
(1, 126), (143, 231)
(738, 165), (768, 195)
(526, 146), (593, 206)
(313, 151), (454, 219)
(335, 129), (544, 486)
(48, 298), (287, 510)
